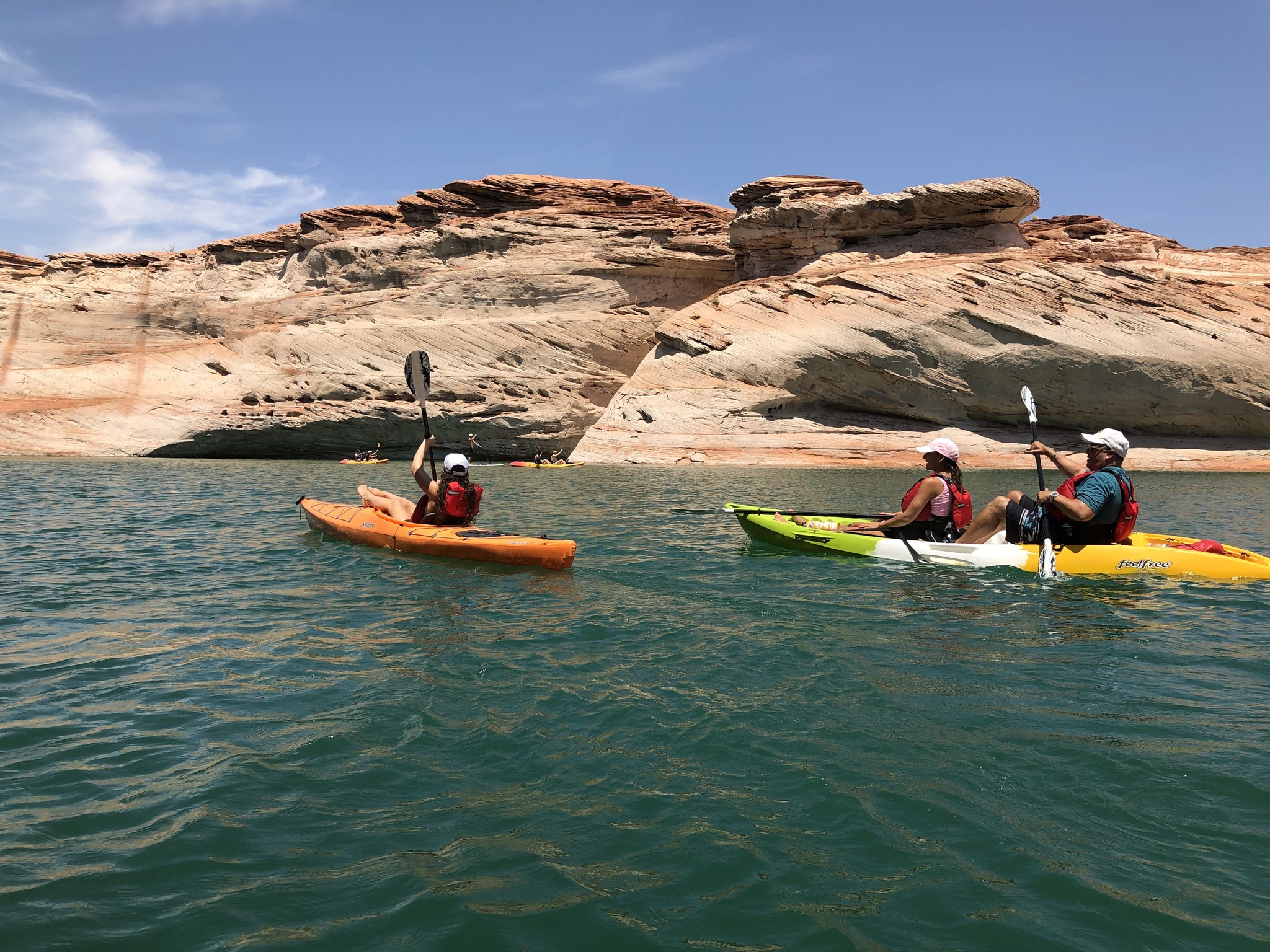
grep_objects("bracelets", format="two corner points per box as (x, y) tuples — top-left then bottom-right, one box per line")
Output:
(882, 520), (885, 528)
(1051, 451), (1056, 462)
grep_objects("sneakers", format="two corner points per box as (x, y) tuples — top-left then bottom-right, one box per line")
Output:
(951, 522), (965, 539)
(925, 530), (951, 543)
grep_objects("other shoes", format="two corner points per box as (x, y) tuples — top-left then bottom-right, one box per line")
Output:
(468, 462), (472, 464)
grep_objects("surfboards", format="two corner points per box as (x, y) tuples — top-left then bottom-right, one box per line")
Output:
(442, 464), (504, 466)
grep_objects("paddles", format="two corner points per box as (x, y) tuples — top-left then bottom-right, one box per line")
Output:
(404, 350), (437, 482)
(476, 436), (492, 464)
(562, 440), (563, 459)
(1021, 385), (1057, 575)
(356, 451), (368, 458)
(375, 443), (380, 464)
(536, 444), (545, 458)
(668, 508), (896, 519)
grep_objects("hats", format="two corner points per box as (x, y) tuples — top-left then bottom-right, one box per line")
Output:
(442, 453), (469, 476)
(916, 438), (960, 462)
(1081, 428), (1129, 458)
(368, 450), (372, 453)
(553, 450), (557, 453)
(469, 433), (473, 436)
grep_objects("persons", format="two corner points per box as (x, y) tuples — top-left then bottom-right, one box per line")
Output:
(551, 449), (566, 465)
(774, 438), (961, 542)
(926, 428), (1132, 545)
(467, 433), (483, 465)
(355, 449), (381, 462)
(357, 435), (483, 526)
(534, 451), (554, 464)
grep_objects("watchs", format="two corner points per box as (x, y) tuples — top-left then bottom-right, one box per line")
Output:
(1049, 491), (1059, 503)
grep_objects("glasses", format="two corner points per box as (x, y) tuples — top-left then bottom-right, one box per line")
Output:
(357, 451), (360, 452)
(1087, 444), (1111, 453)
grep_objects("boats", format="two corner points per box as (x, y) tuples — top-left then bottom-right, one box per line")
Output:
(509, 460), (586, 468)
(722, 502), (1270, 581)
(294, 494), (578, 571)
(339, 459), (390, 465)
